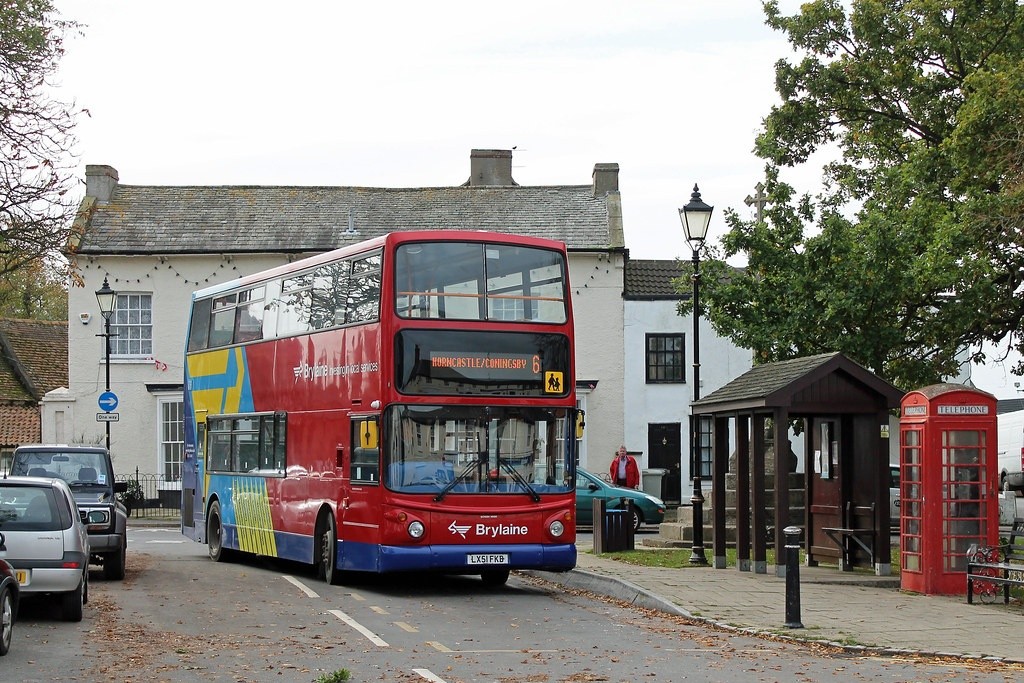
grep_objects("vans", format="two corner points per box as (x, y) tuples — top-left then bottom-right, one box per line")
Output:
(996, 410), (1024, 495)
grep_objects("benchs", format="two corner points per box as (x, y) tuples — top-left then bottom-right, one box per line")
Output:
(965, 517), (1024, 605)
(821, 527), (873, 557)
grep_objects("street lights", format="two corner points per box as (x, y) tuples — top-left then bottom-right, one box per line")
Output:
(94, 277), (119, 449)
(677, 183), (715, 565)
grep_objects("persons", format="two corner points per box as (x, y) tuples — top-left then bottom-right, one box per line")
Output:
(610, 446), (639, 490)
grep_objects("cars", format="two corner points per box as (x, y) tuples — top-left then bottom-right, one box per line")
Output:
(0, 448), (90, 656)
(833, 463), (957, 526)
(481, 465), (667, 532)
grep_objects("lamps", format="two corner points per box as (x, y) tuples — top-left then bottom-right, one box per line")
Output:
(79, 313), (93, 324)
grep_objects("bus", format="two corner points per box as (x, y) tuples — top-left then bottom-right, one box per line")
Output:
(179, 229), (585, 587)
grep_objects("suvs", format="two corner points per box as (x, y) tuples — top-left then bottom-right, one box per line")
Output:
(9, 444), (128, 580)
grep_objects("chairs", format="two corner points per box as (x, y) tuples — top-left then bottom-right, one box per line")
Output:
(28, 468), (47, 477)
(79, 468), (97, 482)
